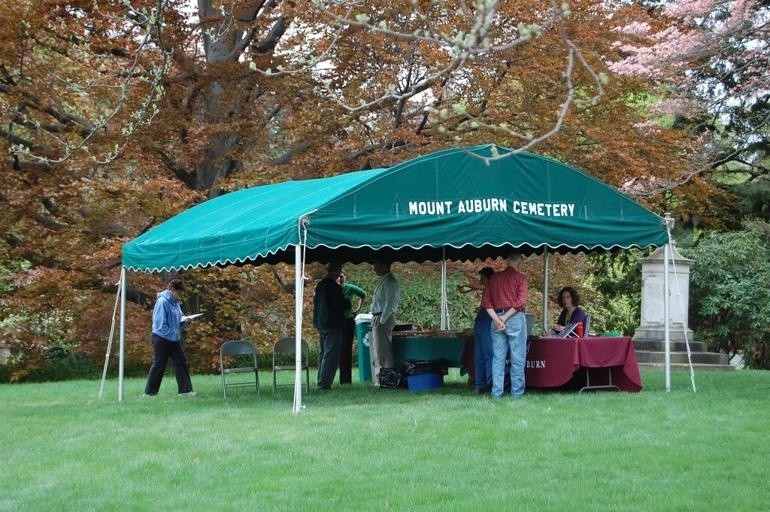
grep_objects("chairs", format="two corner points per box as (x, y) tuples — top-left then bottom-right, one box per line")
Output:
(219, 337), (310, 400)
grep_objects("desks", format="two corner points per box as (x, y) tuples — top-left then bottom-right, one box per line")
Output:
(388, 334), (633, 396)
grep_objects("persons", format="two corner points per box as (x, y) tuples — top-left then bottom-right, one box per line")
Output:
(311, 262), (352, 392)
(142, 279), (198, 397)
(546, 286), (586, 336)
(455, 267), (495, 396)
(482, 252), (528, 398)
(334, 269), (367, 385)
(369, 256), (400, 389)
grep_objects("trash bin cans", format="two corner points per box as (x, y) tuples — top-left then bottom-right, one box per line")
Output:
(404, 359), (444, 391)
